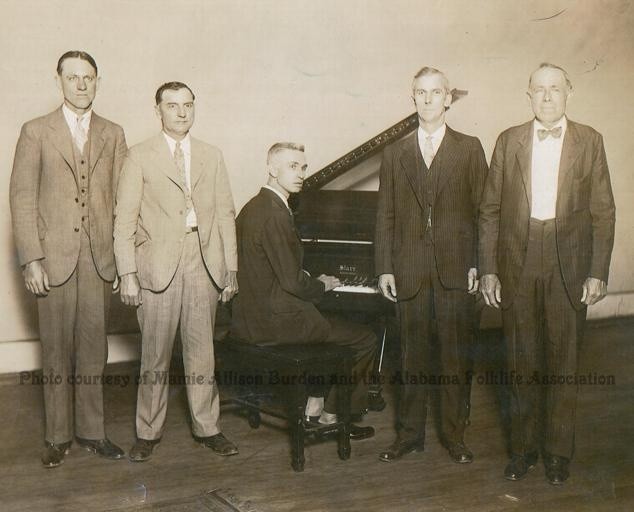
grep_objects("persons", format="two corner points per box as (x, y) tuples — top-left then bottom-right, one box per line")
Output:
(112, 82), (238, 462)
(477, 62), (616, 485)
(372, 67), (490, 461)
(9, 50), (131, 469)
(231, 141), (379, 440)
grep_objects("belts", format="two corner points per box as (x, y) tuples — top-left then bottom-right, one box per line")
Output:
(184, 225), (199, 234)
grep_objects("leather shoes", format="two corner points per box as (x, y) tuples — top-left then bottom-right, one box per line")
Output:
(128, 436), (162, 464)
(378, 435), (426, 464)
(191, 430), (240, 458)
(503, 452), (539, 482)
(74, 434), (127, 461)
(441, 439), (475, 465)
(302, 414), (375, 441)
(364, 388), (388, 413)
(543, 454), (570, 486)
(40, 439), (75, 469)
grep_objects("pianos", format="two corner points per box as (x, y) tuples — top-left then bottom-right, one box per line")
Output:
(284, 89), (483, 411)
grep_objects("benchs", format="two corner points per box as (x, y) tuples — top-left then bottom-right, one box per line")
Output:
(209, 323), (354, 473)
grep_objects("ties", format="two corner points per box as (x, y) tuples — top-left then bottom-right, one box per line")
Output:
(172, 139), (194, 218)
(421, 134), (436, 171)
(73, 115), (89, 156)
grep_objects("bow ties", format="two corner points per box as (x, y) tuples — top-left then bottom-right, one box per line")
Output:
(536, 126), (564, 144)
